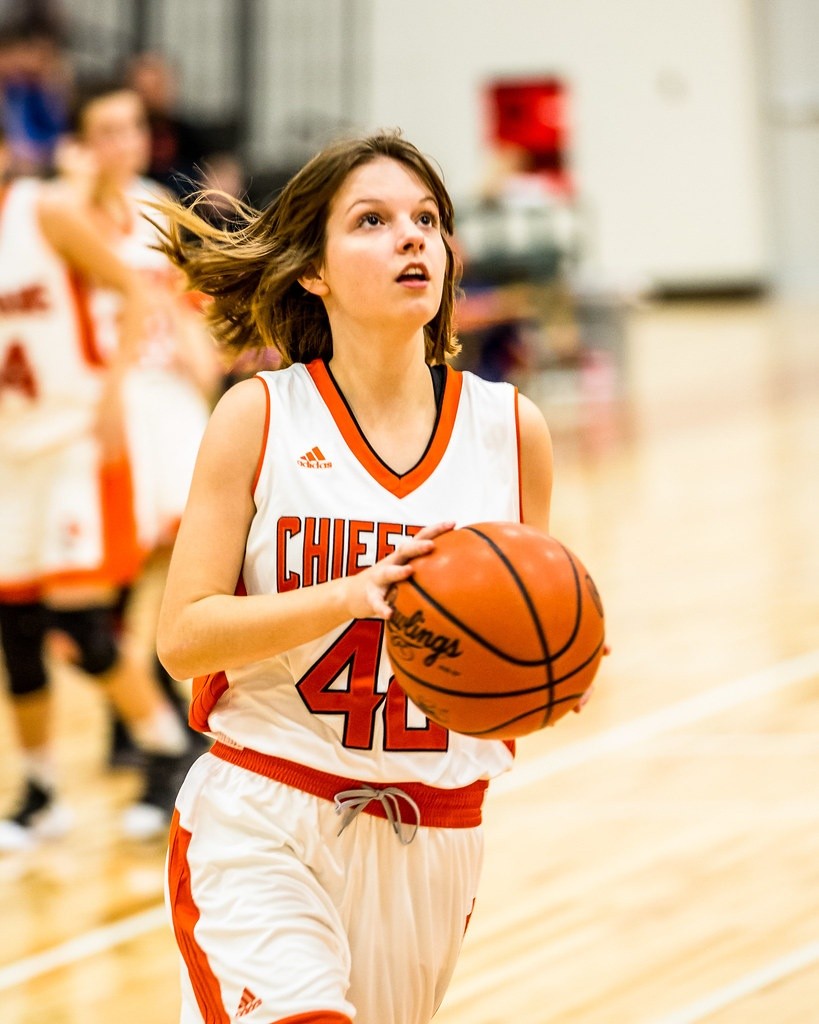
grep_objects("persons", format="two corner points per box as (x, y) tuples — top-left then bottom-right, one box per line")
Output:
(152, 134), (610, 1024)
(0, 1), (282, 850)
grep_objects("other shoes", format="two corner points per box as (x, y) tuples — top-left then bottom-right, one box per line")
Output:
(109, 702), (210, 765)
(121, 749), (191, 844)
(0, 780), (54, 851)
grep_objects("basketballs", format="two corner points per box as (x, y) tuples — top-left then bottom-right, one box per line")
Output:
(385, 517), (607, 741)
